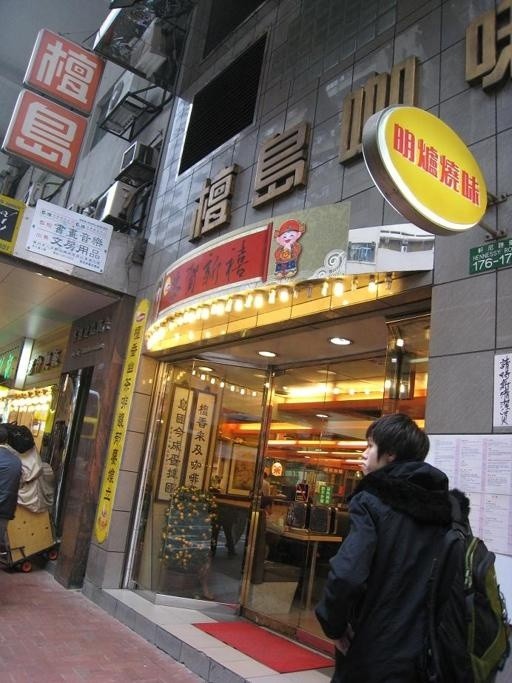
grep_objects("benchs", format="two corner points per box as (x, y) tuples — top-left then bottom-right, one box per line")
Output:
(204, 499), (300, 615)
(307, 505), (351, 602)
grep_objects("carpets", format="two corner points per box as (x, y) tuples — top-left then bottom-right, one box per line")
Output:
(190, 621), (335, 674)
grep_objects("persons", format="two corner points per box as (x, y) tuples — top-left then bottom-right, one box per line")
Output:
(248, 471), (271, 497)
(315, 410), (474, 683)
(1, 424), (24, 552)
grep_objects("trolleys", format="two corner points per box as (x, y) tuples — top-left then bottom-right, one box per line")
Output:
(1, 497), (61, 573)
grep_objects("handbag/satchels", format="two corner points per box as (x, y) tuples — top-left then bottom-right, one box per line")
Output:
(3, 421), (34, 453)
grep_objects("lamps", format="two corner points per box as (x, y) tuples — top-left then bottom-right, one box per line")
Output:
(145, 272), (392, 351)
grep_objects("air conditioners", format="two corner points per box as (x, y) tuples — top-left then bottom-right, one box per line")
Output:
(0, 17), (188, 233)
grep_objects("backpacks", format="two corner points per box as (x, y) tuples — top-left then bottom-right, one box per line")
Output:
(425, 494), (512, 682)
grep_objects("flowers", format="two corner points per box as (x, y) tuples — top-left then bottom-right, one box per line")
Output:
(159, 483), (219, 574)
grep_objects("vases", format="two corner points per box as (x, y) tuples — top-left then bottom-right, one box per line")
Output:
(159, 566), (203, 597)
